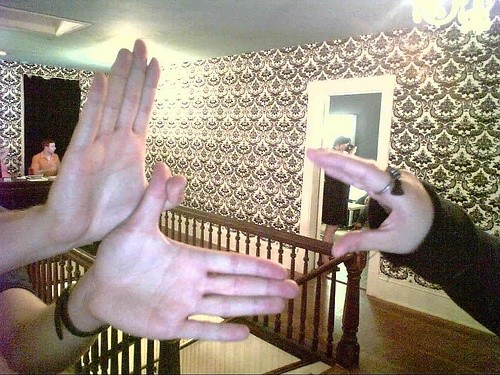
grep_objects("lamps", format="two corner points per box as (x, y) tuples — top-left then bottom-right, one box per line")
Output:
(412, 0), (496, 36)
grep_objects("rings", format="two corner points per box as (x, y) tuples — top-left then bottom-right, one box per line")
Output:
(375, 165), (404, 196)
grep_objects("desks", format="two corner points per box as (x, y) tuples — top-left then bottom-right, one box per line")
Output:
(0, 177), (55, 210)
(348, 203), (364, 227)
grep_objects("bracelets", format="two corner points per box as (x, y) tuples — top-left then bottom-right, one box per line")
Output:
(54, 282), (110, 341)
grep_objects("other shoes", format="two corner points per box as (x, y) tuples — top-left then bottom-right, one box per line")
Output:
(330, 265), (340, 271)
(318, 261), (327, 266)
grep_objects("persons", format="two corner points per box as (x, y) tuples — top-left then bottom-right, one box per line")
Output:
(305, 147), (500, 338)
(0, 39), (299, 375)
(321, 137), (352, 271)
(31, 138), (61, 176)
